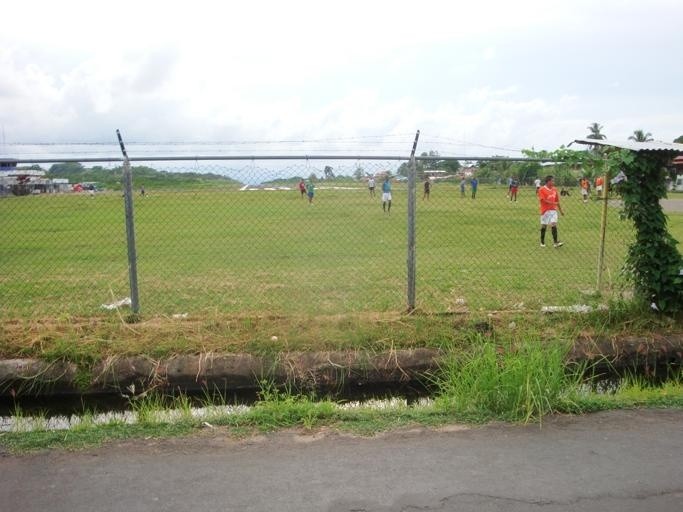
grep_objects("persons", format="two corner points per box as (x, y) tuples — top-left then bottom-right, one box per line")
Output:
(367, 175), (379, 198)
(504, 175), (514, 198)
(536, 174), (565, 249)
(578, 175), (626, 202)
(139, 182), (145, 195)
(470, 176), (477, 199)
(304, 181), (315, 207)
(559, 186), (571, 196)
(298, 177), (306, 197)
(509, 177), (518, 201)
(534, 177), (540, 197)
(459, 175), (465, 197)
(381, 176), (392, 213)
(420, 177), (432, 202)
(88, 183), (96, 201)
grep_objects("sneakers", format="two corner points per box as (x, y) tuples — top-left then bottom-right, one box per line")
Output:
(540, 242), (545, 247)
(554, 243), (563, 247)
